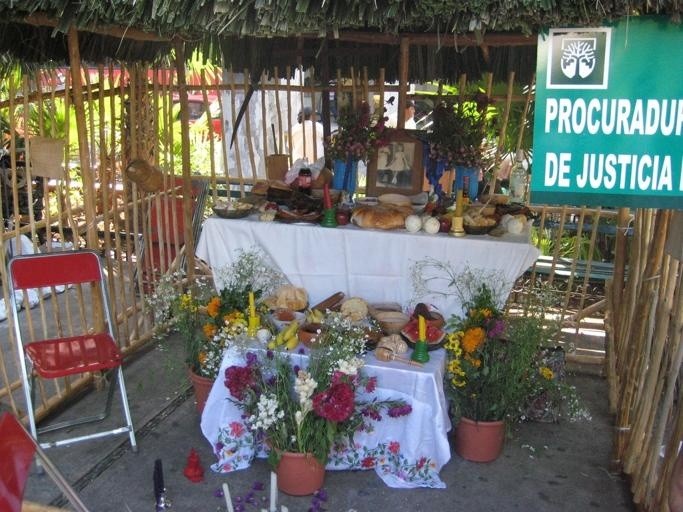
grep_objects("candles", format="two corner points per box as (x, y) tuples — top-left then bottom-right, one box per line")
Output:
(418, 312), (427, 342)
(248, 289), (256, 315)
(323, 181), (332, 209)
(456, 189), (463, 217)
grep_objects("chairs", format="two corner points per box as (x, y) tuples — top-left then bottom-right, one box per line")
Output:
(7, 250), (138, 476)
(133, 176), (211, 300)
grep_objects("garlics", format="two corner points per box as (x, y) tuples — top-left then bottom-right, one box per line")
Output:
(424, 217), (441, 233)
(506, 216), (524, 234)
(404, 214), (422, 232)
(500, 214), (511, 230)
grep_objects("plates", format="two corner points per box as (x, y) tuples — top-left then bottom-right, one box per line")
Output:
(463, 224), (496, 235)
(354, 196), (380, 206)
(412, 202), (425, 211)
(212, 206), (253, 218)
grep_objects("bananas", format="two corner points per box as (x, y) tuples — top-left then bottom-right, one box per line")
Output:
(266, 319), (301, 350)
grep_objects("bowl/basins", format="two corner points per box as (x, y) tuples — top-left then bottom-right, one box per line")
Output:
(470, 205), (496, 217)
(377, 312), (410, 335)
(412, 311), (445, 328)
(373, 303), (402, 313)
(479, 194), (511, 205)
(299, 323), (331, 349)
(271, 312), (306, 332)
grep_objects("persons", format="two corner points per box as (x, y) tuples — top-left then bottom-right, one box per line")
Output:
(287, 107), (325, 166)
(389, 144), (411, 184)
(405, 101), (417, 130)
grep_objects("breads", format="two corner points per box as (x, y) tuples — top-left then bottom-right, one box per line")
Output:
(349, 203), (415, 229)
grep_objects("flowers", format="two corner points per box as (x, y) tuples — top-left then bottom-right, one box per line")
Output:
(406, 255), (590, 424)
(223, 309), (412, 450)
(425, 100), (485, 166)
(333, 154), (356, 194)
(143, 244), (282, 395)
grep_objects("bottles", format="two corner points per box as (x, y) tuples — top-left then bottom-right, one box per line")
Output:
(462, 175), (469, 205)
(299, 156), (312, 196)
(509, 149), (528, 203)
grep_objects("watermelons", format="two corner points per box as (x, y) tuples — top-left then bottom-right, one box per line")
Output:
(400, 311), (449, 351)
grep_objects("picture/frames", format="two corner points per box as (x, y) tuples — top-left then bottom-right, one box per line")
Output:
(367, 129), (425, 200)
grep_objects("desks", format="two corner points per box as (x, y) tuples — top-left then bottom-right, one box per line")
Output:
(196, 212), (542, 327)
(198, 316), (450, 490)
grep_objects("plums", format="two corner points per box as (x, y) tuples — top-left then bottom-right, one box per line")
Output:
(335, 211), (351, 224)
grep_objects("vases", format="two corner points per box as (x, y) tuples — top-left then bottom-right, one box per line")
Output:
(266, 443), (324, 496)
(322, 97), (388, 165)
(188, 361), (216, 414)
(438, 157), (478, 201)
(452, 409), (508, 463)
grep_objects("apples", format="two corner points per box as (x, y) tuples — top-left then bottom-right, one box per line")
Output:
(440, 217), (450, 233)
